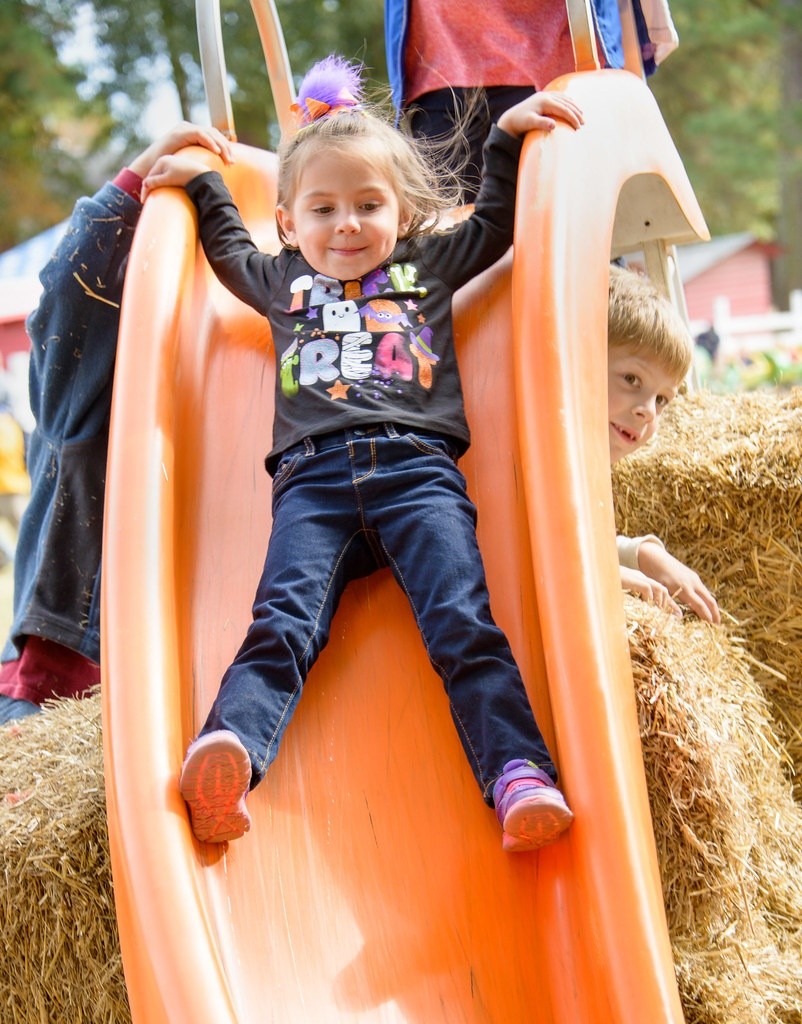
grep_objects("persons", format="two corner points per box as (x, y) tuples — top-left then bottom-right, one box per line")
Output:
(385, 0), (679, 206)
(0, 121), (237, 726)
(140, 52), (586, 851)
(608, 263), (721, 623)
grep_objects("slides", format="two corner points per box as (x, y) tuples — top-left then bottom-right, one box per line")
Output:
(93, 64), (716, 1024)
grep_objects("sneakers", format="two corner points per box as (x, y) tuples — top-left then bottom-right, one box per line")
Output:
(491, 758), (576, 846)
(180, 730), (252, 843)
